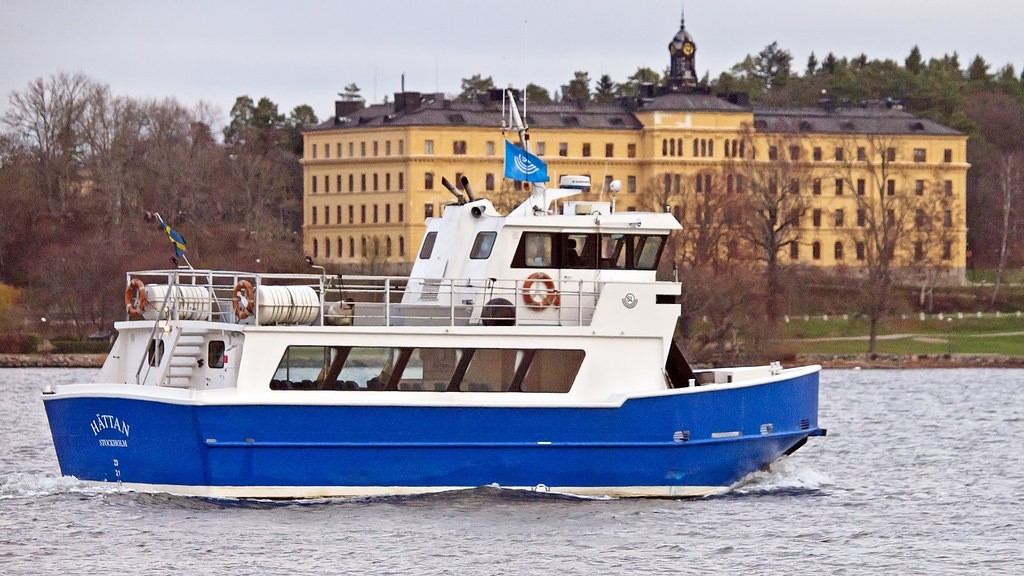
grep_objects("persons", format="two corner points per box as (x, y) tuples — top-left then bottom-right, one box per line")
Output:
(568, 239), (577, 262)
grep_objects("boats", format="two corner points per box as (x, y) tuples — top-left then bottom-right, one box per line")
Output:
(39, 19), (830, 504)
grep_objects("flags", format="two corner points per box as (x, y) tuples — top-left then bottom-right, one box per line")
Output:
(162, 222), (187, 256)
(504, 140), (550, 183)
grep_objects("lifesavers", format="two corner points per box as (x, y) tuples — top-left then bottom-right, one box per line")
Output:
(124, 278), (146, 317)
(232, 280), (255, 319)
(523, 272), (555, 312)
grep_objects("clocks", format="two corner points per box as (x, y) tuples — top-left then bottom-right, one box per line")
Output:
(682, 42), (695, 56)
